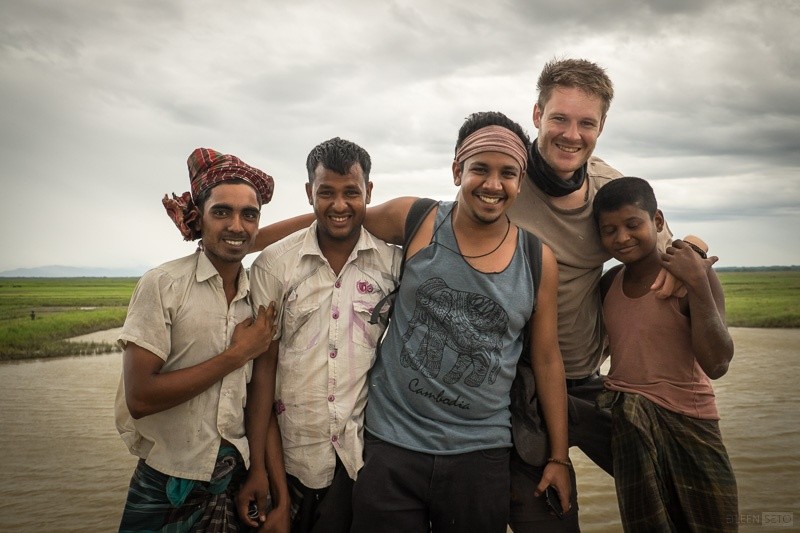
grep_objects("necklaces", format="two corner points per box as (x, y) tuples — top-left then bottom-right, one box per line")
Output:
(430, 201), (511, 260)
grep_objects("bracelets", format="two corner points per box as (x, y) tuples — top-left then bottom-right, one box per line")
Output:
(547, 455), (573, 468)
(685, 240), (708, 259)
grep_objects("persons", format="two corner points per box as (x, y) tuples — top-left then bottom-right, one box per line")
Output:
(589, 174), (737, 533)
(234, 108), (578, 532)
(504, 54), (713, 475)
(111, 142), (290, 533)
(244, 135), (402, 531)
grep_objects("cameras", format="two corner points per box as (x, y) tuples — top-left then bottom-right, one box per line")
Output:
(538, 485), (565, 520)
